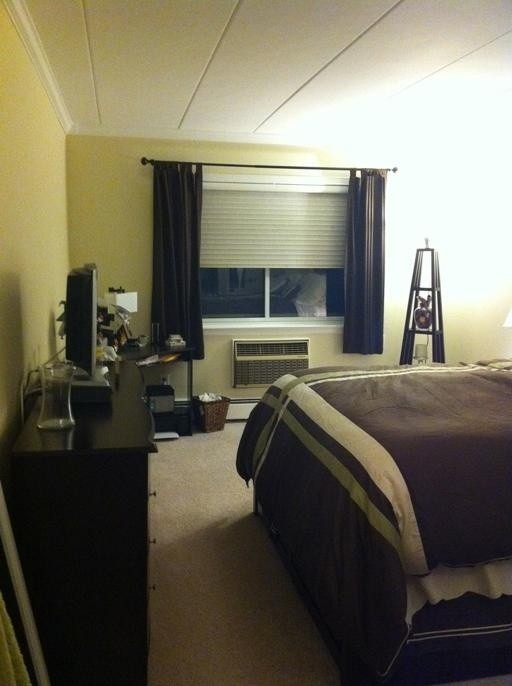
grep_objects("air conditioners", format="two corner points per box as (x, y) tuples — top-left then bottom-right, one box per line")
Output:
(232, 339), (309, 388)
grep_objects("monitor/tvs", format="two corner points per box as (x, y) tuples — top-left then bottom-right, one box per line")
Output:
(64, 261), (98, 377)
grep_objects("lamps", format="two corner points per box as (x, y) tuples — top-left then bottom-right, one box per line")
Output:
(413, 344), (428, 364)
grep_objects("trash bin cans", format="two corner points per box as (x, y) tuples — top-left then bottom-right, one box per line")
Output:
(193, 394), (230, 433)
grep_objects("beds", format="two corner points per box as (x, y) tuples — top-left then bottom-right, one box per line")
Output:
(252, 362), (512, 685)
